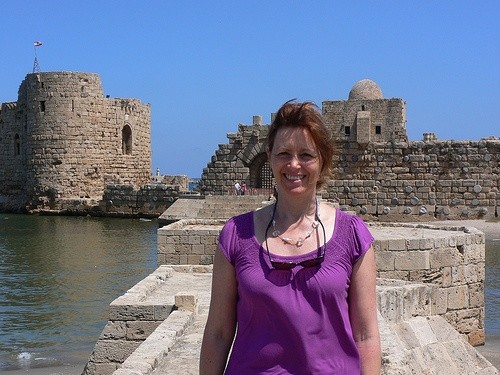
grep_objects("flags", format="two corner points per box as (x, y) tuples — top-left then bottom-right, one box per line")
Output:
(33, 40), (43, 47)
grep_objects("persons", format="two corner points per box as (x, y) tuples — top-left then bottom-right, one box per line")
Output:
(198, 96), (383, 375)
(240, 181), (247, 194)
(234, 181), (241, 195)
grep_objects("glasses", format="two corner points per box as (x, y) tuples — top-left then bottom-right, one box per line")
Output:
(265, 216), (327, 270)
